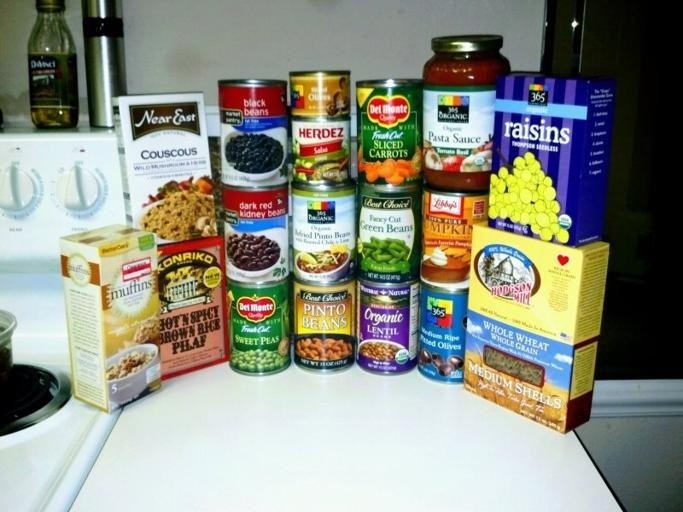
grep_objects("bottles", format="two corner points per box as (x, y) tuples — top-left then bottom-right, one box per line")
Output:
(420, 32), (508, 194)
(80, 0), (129, 130)
(23, 0), (80, 130)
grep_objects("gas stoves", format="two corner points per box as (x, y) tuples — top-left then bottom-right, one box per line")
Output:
(0, 270), (129, 511)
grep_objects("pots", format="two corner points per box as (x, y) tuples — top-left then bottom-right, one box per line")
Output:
(0, 307), (15, 381)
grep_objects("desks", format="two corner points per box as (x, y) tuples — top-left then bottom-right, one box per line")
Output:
(33, 352), (629, 512)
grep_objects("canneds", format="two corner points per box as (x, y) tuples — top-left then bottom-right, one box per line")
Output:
(217, 79), (290, 376)
(355, 78), (424, 376)
(416, 35), (510, 381)
(289, 70), (358, 372)
